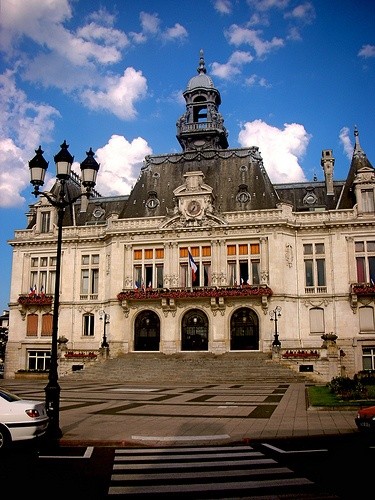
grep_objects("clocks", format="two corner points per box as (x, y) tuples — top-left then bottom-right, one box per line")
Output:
(187, 201), (201, 215)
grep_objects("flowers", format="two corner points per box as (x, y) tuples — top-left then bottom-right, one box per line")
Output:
(283, 351), (320, 358)
(18, 293), (53, 304)
(64, 352), (97, 359)
(117, 284), (273, 301)
(354, 286), (375, 293)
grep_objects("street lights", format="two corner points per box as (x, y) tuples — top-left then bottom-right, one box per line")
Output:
(28, 140), (101, 454)
(99, 309), (110, 362)
(270, 306), (281, 362)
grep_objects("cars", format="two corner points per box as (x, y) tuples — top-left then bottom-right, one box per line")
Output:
(355, 405), (375, 446)
(0, 389), (49, 449)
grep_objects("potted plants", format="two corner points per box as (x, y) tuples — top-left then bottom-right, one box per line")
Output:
(15, 369), (49, 379)
(57, 335), (68, 344)
(321, 331), (338, 341)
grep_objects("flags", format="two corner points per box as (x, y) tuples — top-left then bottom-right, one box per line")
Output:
(40, 283), (45, 298)
(188, 249), (198, 282)
(29, 284), (37, 296)
